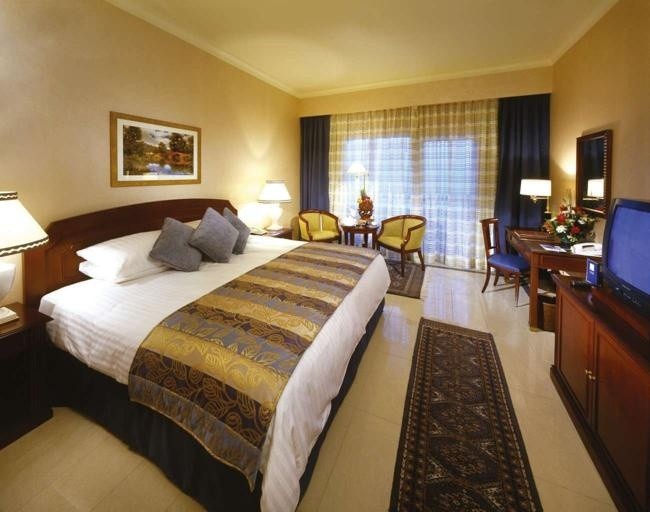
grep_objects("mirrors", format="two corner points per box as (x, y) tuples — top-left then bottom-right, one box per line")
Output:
(575, 130), (612, 219)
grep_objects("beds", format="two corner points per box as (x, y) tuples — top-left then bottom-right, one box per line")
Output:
(24, 199), (391, 511)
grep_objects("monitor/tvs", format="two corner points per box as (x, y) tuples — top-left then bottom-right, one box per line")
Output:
(602, 198), (650, 315)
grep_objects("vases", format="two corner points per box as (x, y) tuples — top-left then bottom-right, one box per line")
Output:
(559, 242), (577, 248)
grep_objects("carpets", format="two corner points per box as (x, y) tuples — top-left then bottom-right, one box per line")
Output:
(385, 259), (426, 300)
(388, 315), (543, 512)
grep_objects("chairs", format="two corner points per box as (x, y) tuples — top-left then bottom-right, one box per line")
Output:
(480, 218), (530, 307)
(298, 209), (342, 244)
(376, 214), (427, 277)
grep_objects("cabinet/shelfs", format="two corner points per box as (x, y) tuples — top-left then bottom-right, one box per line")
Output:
(550, 277), (649, 512)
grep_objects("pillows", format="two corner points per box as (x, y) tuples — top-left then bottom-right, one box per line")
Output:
(77, 230), (167, 278)
(79, 262), (166, 283)
(149, 216), (204, 272)
(222, 207), (249, 255)
(186, 220), (200, 228)
(188, 206), (239, 263)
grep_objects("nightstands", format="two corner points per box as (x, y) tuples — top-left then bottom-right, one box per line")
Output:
(0, 302), (53, 450)
(251, 228), (291, 239)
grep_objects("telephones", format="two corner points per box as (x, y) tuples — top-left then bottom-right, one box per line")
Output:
(248, 225), (266, 235)
(570, 242), (603, 258)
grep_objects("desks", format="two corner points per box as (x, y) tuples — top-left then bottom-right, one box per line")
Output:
(341, 224), (379, 249)
(506, 228), (602, 332)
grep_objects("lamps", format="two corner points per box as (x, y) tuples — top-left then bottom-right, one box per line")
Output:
(519, 178), (552, 220)
(0, 190), (50, 325)
(346, 161), (368, 177)
(582, 179), (604, 201)
(258, 181), (292, 231)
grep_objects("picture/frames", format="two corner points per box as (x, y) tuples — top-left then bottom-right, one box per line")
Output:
(108, 111), (202, 187)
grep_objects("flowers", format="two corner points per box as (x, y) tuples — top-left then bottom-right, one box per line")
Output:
(541, 189), (596, 243)
